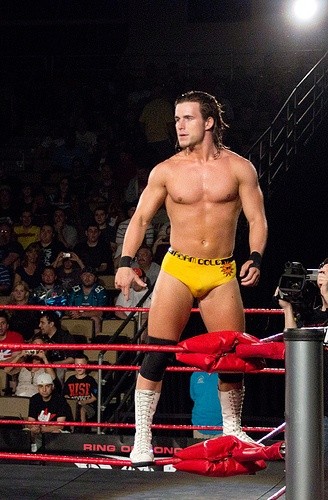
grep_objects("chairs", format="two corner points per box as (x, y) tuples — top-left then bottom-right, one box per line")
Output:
(0, 276), (136, 423)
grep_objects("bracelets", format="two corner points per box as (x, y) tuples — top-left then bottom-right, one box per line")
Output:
(118, 256), (133, 268)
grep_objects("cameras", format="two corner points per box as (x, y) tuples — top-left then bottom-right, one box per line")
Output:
(27, 350), (37, 355)
(62, 253), (71, 258)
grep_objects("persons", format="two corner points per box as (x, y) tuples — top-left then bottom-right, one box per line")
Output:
(0, 52), (295, 464)
(279, 260), (328, 471)
(189, 362), (226, 440)
(113, 89), (270, 465)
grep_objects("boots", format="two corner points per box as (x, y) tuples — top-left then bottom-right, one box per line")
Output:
(218, 386), (265, 447)
(130, 390), (161, 463)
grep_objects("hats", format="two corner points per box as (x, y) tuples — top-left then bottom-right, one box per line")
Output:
(36, 373), (53, 385)
(81, 266), (97, 277)
